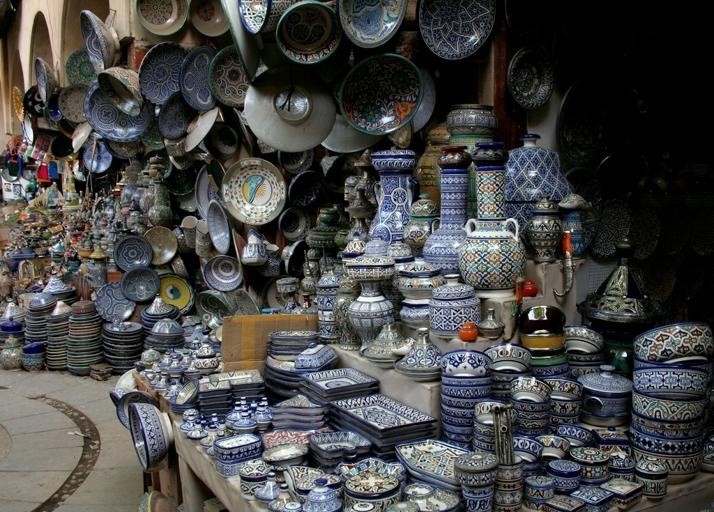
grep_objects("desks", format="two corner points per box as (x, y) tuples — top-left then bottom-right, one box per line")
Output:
(172, 420), (268, 511)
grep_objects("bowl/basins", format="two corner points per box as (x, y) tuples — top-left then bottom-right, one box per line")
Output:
(124, 403), (173, 470)
(113, 391), (158, 430)
(438, 321), (713, 507)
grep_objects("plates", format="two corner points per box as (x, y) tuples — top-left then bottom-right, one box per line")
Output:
(557, 84), (609, 167)
(24, 226), (193, 373)
(10, 3), (498, 290)
(505, 45), (556, 109)
(580, 203), (660, 262)
(176, 331), (475, 511)
